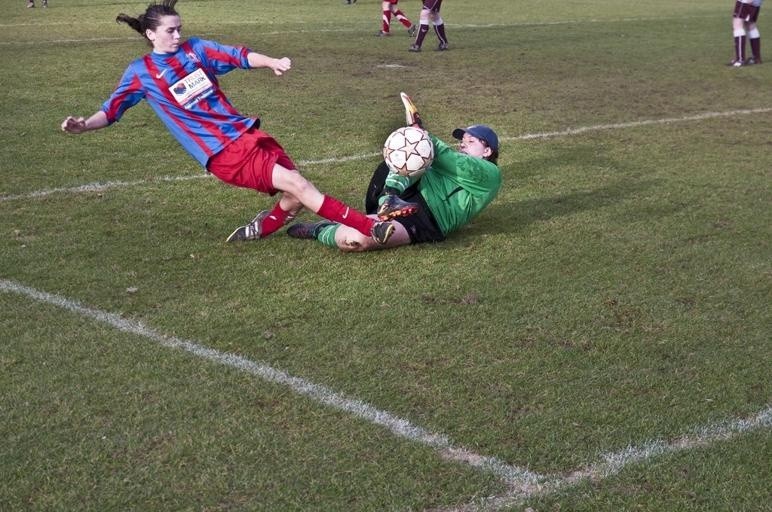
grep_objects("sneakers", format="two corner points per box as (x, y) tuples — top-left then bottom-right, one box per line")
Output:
(377, 194), (420, 221)
(437, 43), (448, 51)
(728, 56), (761, 67)
(407, 24), (420, 51)
(287, 223), (318, 240)
(375, 30), (390, 37)
(226, 210), (268, 243)
(372, 221), (395, 248)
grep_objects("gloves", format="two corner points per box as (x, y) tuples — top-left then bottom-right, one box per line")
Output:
(400, 92), (423, 129)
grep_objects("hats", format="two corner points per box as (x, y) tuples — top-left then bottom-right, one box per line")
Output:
(452, 125), (498, 151)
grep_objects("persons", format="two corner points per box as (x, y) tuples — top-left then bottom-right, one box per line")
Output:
(409, 0), (448, 52)
(27, 0), (48, 8)
(61, 0), (395, 245)
(725, 0), (764, 67)
(287, 92), (502, 252)
(373, 0), (419, 37)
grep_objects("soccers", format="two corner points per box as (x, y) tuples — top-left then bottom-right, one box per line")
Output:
(382, 127), (434, 176)
